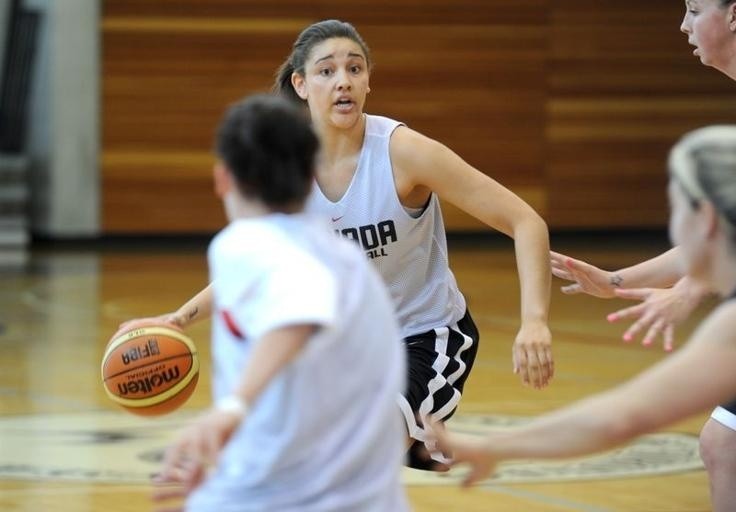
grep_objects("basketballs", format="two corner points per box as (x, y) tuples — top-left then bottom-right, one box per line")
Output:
(101, 319), (199, 417)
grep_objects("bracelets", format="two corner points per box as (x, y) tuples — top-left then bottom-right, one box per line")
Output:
(217, 395), (248, 423)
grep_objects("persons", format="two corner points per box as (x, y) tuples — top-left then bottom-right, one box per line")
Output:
(421, 126), (736, 512)
(550, 0), (736, 512)
(149, 95), (407, 512)
(118, 19), (555, 471)
